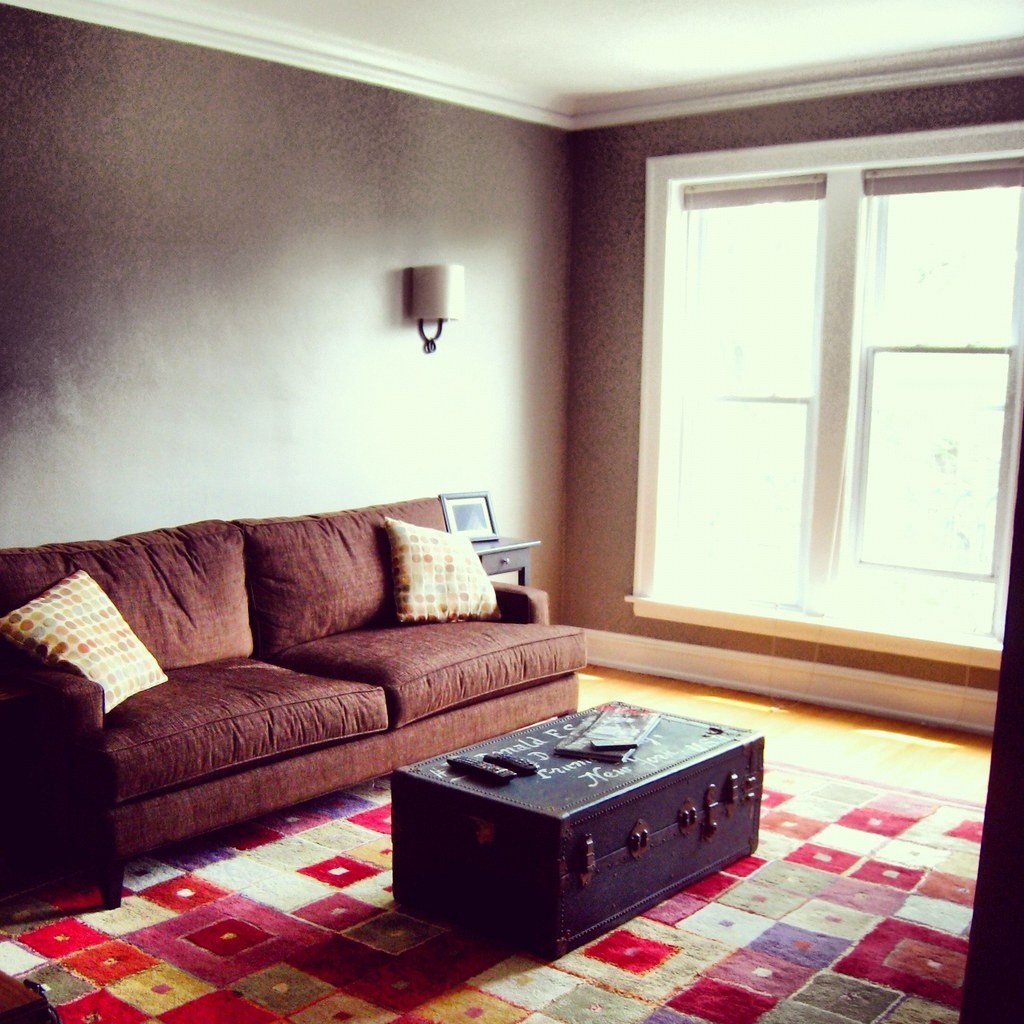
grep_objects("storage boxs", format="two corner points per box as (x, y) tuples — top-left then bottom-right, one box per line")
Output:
(390, 700), (765, 963)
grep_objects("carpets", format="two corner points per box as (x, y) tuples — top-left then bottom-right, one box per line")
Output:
(0, 762), (990, 1024)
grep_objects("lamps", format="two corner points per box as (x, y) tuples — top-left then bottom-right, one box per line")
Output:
(410, 265), (464, 354)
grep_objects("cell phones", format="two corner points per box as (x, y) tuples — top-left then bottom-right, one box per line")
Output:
(591, 739), (638, 751)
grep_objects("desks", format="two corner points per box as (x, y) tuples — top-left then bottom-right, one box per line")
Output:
(471, 537), (542, 586)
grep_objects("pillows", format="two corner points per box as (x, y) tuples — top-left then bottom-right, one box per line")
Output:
(383, 516), (502, 622)
(0, 570), (168, 714)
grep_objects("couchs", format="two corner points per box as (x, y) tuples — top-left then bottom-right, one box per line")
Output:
(0, 497), (588, 911)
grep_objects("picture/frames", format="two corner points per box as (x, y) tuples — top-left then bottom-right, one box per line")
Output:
(438, 492), (499, 542)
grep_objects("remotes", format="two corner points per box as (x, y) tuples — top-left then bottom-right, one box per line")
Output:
(447, 756), (518, 782)
(483, 753), (538, 774)
